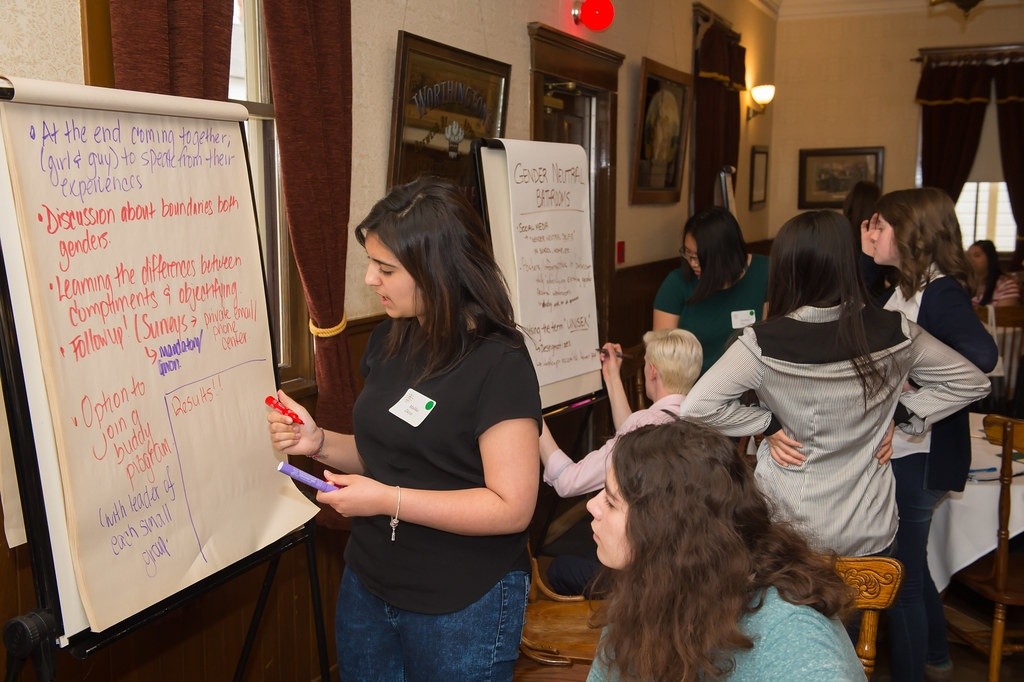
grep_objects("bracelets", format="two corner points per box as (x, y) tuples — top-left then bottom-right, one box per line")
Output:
(305, 427), (325, 459)
(390, 486), (401, 541)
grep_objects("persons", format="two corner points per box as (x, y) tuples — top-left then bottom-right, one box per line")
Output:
(267, 176), (544, 682)
(539, 181), (1019, 682)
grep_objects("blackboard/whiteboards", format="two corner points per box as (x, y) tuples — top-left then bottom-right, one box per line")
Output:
(0, 76), (314, 659)
(472, 137), (613, 426)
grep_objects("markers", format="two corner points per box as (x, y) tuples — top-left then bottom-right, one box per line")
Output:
(278, 461), (338, 493)
(264, 396), (304, 425)
(542, 405), (567, 419)
(595, 348), (632, 361)
(571, 396), (598, 406)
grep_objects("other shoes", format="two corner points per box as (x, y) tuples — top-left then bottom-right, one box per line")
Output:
(922, 659), (954, 674)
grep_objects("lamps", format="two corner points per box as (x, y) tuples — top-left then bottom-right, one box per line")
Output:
(746, 85), (776, 121)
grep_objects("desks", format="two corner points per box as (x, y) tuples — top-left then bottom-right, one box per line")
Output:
(924, 409), (1024, 594)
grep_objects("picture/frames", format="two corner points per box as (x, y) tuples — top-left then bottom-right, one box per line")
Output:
(626, 57), (693, 206)
(390, 29), (511, 226)
(750, 144), (770, 213)
(796, 144), (887, 210)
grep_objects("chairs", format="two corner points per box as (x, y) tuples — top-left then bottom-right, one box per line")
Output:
(519, 303), (1024, 680)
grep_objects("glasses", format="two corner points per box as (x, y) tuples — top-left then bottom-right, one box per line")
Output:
(679, 246), (702, 266)
